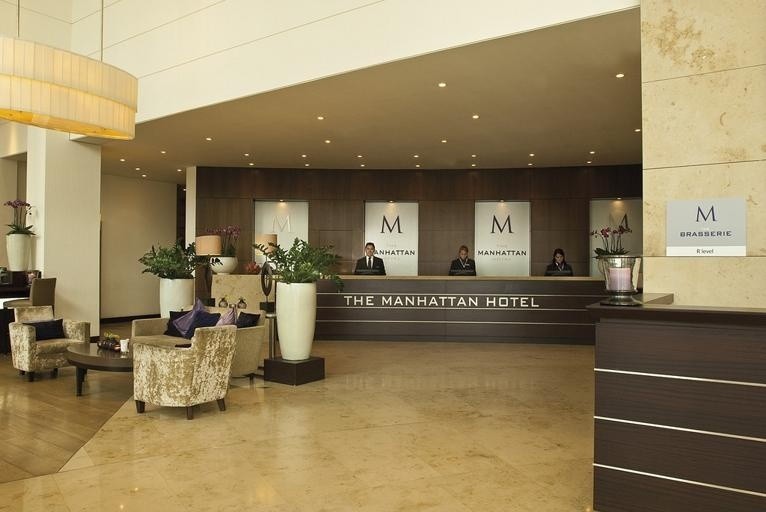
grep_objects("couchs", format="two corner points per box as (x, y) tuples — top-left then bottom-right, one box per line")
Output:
(130, 306), (266, 385)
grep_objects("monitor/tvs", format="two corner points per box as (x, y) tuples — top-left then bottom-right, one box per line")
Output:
(546, 269), (571, 276)
(355, 269), (383, 275)
(451, 270), (475, 276)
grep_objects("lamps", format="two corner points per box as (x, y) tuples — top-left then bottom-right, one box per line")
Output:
(0, 0), (138, 141)
(255, 234), (277, 313)
(196, 235), (221, 306)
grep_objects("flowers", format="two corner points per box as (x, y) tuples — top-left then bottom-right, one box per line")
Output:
(590, 226), (632, 255)
(244, 262), (260, 274)
(4, 200), (37, 235)
(205, 225), (241, 257)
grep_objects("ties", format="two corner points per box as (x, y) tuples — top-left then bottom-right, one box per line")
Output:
(368, 258), (371, 269)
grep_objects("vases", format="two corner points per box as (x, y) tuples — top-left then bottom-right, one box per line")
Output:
(210, 256), (238, 275)
(6, 233), (30, 272)
(598, 256), (641, 306)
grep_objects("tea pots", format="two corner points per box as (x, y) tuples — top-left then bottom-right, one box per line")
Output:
(109, 341), (120, 351)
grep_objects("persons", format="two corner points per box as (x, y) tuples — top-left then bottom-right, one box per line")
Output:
(544, 248), (572, 276)
(355, 242), (386, 275)
(447, 245), (475, 275)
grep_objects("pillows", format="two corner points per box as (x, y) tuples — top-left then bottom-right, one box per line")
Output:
(22, 319), (65, 340)
(163, 297), (260, 340)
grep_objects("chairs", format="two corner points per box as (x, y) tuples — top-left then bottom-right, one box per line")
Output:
(9, 306), (90, 382)
(132, 324), (237, 420)
(0, 266), (56, 316)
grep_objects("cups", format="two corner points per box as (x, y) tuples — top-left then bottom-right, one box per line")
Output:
(119, 352), (128, 359)
(120, 340), (129, 352)
(228, 303), (236, 307)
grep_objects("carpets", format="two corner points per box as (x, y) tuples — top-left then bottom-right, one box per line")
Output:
(1, 320), (133, 483)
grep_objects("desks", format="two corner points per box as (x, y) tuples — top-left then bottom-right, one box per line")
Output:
(266, 313), (277, 359)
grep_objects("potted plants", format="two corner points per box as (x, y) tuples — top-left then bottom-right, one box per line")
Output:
(252, 237), (344, 361)
(138, 242), (196, 318)
